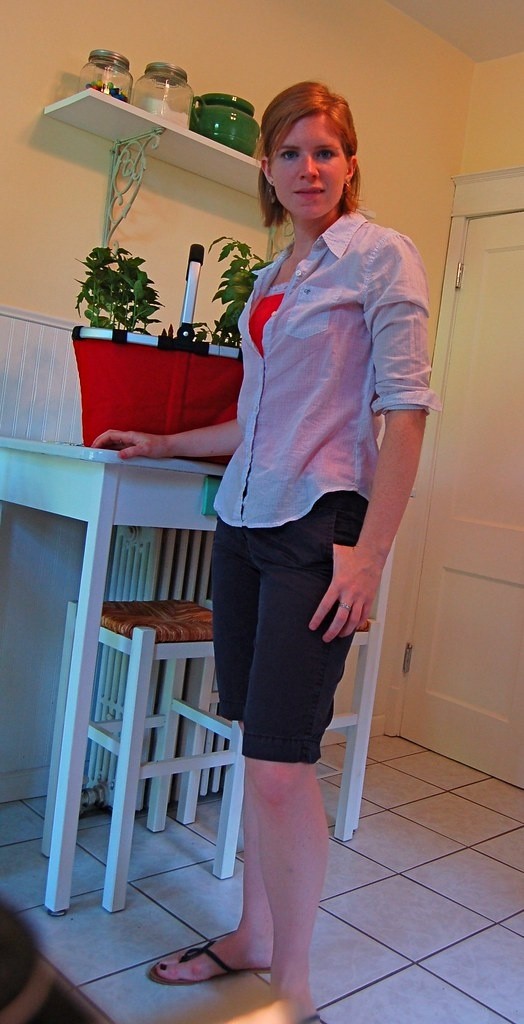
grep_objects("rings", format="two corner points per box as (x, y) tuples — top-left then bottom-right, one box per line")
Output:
(339, 602), (352, 612)
(119, 439), (124, 446)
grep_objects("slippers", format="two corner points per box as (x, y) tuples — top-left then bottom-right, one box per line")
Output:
(147, 939), (272, 985)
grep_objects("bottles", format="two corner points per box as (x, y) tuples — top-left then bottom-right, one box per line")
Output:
(79, 49), (133, 105)
(132, 61), (194, 131)
(190, 92), (261, 158)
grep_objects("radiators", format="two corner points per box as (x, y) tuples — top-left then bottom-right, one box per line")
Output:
(84, 525), (242, 812)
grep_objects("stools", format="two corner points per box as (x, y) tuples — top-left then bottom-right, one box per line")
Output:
(42, 596), (372, 916)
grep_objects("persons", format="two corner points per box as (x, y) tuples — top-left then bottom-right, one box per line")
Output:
(89, 80), (432, 1023)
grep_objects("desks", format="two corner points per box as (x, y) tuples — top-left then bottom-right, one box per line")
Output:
(0, 435), (396, 920)
